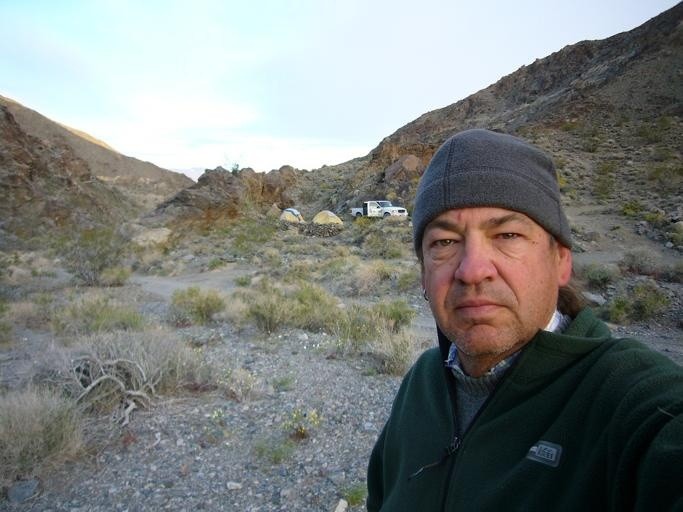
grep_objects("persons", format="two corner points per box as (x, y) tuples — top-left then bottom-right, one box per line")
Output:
(366, 128), (683, 512)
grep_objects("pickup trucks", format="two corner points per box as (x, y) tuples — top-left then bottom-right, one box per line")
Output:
(349, 199), (407, 220)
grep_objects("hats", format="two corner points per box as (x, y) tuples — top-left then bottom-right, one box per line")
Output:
(414, 129), (572, 254)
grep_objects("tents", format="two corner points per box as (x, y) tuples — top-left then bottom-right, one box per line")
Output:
(280, 208), (306, 224)
(311, 209), (343, 225)
